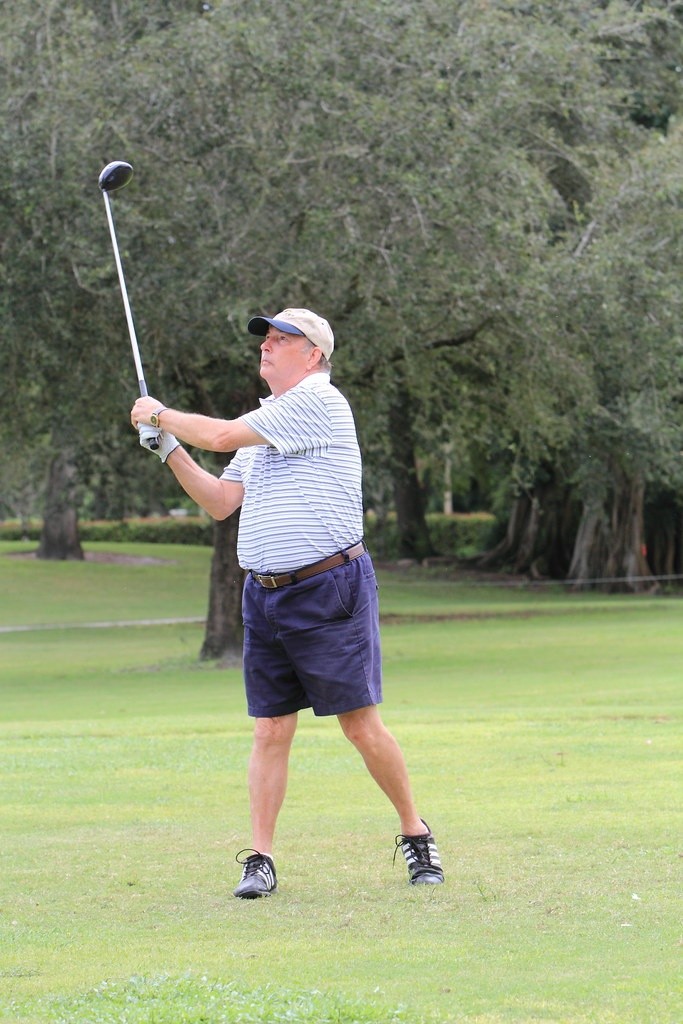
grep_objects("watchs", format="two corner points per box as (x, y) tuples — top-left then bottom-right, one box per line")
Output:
(150, 408), (169, 428)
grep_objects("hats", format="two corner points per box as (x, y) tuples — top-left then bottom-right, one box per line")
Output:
(248, 308), (334, 362)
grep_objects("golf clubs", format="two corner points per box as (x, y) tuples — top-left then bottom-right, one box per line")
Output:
(99, 158), (165, 449)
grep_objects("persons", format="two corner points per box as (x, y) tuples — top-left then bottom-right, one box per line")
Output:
(131, 307), (444, 899)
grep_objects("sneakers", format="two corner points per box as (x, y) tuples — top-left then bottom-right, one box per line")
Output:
(393, 819), (445, 884)
(233, 849), (279, 898)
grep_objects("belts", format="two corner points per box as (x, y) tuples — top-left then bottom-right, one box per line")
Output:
(248, 542), (365, 589)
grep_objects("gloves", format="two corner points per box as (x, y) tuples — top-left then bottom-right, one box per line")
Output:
(137, 421), (180, 463)
